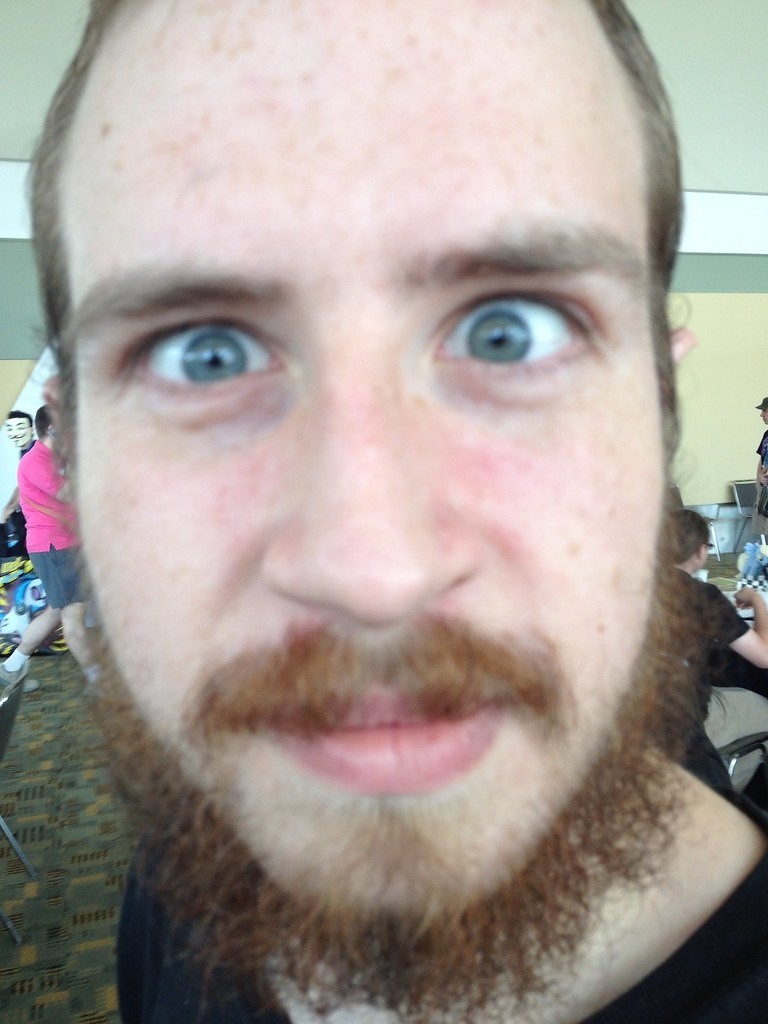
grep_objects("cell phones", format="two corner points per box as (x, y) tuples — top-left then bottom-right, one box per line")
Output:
(47, 429), (56, 438)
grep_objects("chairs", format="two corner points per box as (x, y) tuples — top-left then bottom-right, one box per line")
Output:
(689, 480), (757, 563)
(0, 659), (38, 946)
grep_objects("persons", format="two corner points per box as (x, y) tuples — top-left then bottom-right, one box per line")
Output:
(0, 404), (106, 709)
(752, 395), (768, 538)
(1, 0), (766, 1024)
(643, 509), (768, 789)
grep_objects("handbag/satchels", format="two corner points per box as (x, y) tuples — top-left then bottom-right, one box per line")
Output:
(758, 485), (768, 518)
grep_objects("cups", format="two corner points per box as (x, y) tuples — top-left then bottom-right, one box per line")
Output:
(689, 569), (709, 583)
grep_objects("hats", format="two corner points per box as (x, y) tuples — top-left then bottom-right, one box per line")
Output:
(755, 397), (768, 410)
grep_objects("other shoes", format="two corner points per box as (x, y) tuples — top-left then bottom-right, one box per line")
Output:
(86, 680), (106, 698)
(0, 663), (40, 693)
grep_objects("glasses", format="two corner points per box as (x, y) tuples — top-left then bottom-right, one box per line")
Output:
(701, 542), (713, 549)
